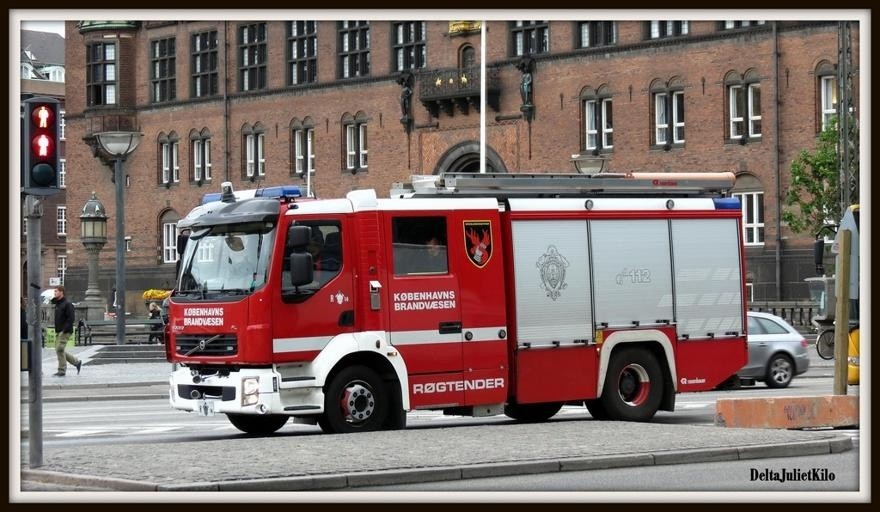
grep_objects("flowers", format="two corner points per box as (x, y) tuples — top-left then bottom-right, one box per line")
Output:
(142, 289), (169, 299)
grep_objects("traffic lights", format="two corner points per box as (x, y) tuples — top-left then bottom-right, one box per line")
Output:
(20, 96), (65, 198)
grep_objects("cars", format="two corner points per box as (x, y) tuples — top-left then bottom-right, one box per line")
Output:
(729, 308), (811, 389)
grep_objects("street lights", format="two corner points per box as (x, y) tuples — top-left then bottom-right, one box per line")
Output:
(569, 155), (612, 174)
(93, 131), (146, 346)
(78, 189), (107, 321)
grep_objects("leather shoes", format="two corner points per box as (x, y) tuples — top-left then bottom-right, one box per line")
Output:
(54, 372), (65, 377)
(76, 360), (81, 373)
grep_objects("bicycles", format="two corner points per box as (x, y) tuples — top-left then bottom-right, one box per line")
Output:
(815, 321), (848, 363)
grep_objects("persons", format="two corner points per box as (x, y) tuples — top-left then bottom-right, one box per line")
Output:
(309, 230), (334, 291)
(147, 302), (164, 345)
(418, 237), (447, 274)
(51, 285), (82, 377)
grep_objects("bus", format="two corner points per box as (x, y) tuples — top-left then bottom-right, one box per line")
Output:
(812, 205), (859, 386)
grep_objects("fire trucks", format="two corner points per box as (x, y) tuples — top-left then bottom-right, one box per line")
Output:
(165, 156), (753, 431)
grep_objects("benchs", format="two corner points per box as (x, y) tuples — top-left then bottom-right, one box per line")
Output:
(72, 318), (168, 349)
